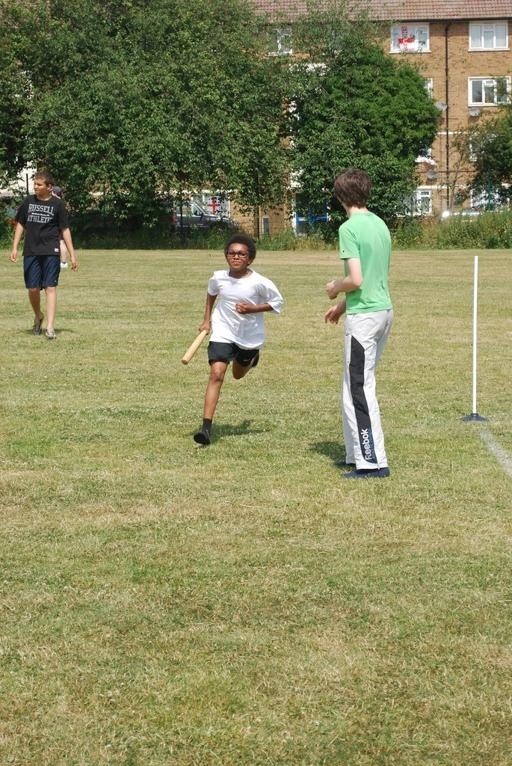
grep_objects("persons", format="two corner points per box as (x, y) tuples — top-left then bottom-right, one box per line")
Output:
(51, 185), (68, 268)
(194, 236), (284, 445)
(323, 170), (395, 478)
(9, 173), (78, 339)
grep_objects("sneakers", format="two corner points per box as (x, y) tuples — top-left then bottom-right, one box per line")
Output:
(342, 467), (389, 479)
(194, 432), (210, 444)
(334, 461), (356, 467)
(45, 327), (56, 339)
(32, 313), (44, 335)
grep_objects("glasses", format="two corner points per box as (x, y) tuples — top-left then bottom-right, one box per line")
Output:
(226, 251), (248, 257)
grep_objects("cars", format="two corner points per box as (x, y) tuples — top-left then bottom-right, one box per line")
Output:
(176, 199), (235, 231)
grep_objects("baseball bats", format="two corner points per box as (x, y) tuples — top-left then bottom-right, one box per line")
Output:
(182, 329), (207, 365)
